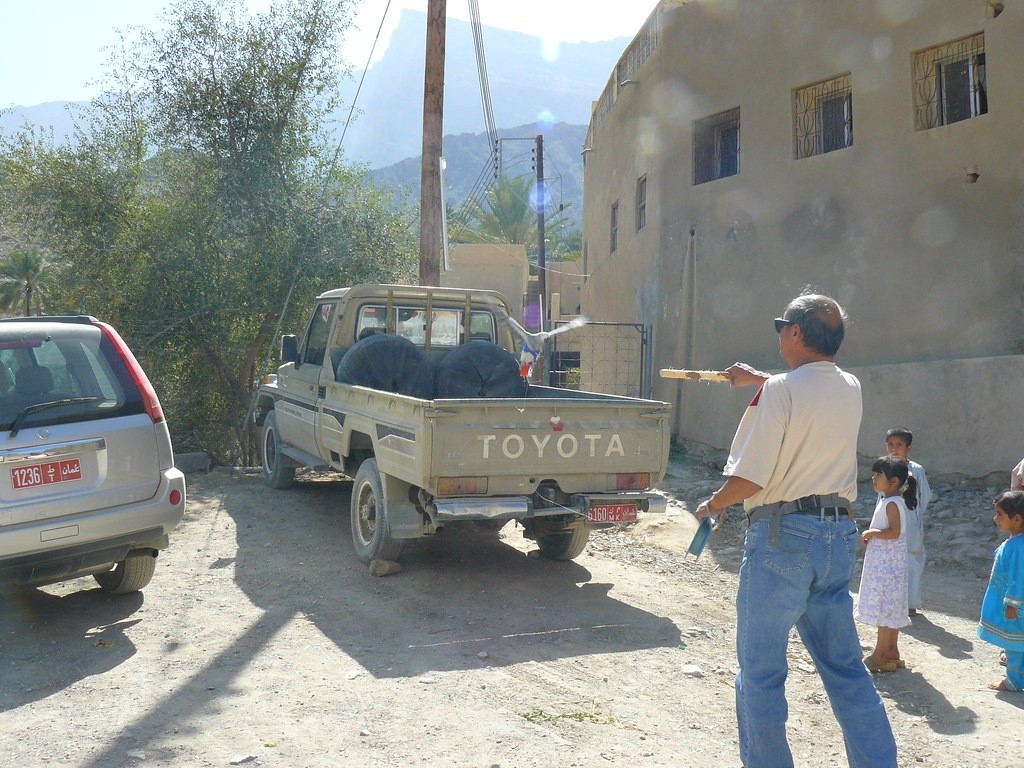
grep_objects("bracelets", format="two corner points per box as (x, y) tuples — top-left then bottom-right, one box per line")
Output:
(706, 499), (725, 517)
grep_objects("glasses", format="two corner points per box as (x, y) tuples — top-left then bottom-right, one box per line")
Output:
(774, 317), (796, 333)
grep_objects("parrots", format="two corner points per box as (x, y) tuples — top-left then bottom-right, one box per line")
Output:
(481, 294), (586, 382)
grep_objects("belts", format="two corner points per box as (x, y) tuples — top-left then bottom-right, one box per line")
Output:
(790, 507), (848, 516)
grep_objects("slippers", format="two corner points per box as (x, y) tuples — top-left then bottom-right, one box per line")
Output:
(863, 656), (897, 672)
(888, 659), (905, 668)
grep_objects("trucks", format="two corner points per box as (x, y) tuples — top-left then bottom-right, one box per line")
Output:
(251, 281), (674, 568)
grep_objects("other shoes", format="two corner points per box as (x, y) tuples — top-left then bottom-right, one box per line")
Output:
(998, 649), (1007, 666)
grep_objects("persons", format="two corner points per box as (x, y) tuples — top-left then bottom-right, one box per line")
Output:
(695, 294), (896, 767)
(861, 456), (916, 673)
(977, 458), (1024, 694)
(885, 426), (932, 617)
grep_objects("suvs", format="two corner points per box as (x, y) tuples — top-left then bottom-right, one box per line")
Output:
(0, 315), (189, 597)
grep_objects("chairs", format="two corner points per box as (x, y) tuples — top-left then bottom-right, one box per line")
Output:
(359, 327), (386, 339)
(16, 366), (63, 414)
(460, 332), (491, 345)
